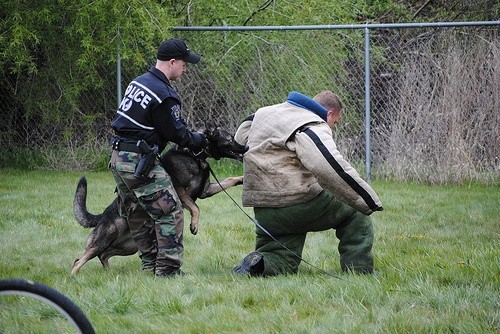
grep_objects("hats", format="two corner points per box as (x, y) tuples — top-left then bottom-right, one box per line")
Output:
(157, 38), (203, 65)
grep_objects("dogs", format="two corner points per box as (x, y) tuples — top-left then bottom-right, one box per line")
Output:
(70, 121), (252, 277)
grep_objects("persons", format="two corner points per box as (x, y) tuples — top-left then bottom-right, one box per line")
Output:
(233, 89), (384, 275)
(110, 37), (217, 277)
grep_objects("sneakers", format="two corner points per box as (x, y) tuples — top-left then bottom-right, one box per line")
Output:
(229, 249), (267, 282)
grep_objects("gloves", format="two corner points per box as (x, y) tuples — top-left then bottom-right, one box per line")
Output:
(195, 132), (206, 154)
